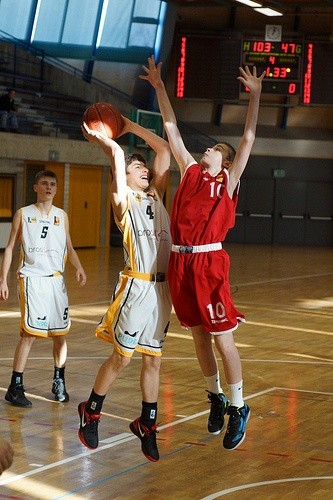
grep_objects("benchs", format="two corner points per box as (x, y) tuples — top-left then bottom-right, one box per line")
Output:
(0, 68), (129, 143)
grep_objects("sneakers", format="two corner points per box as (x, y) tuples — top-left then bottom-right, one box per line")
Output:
(78, 401), (102, 450)
(51, 377), (70, 402)
(222, 402), (251, 451)
(129, 417), (160, 463)
(208, 393), (230, 436)
(5, 384), (33, 407)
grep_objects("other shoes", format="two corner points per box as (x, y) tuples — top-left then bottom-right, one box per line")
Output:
(0, 127), (22, 134)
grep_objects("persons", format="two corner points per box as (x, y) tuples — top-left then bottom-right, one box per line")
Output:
(78, 115), (172, 462)
(0, 171), (87, 408)
(0, 89), (22, 133)
(139, 55), (266, 451)
(0, 435), (14, 476)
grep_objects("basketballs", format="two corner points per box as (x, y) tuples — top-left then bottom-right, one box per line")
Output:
(81, 101), (124, 140)
